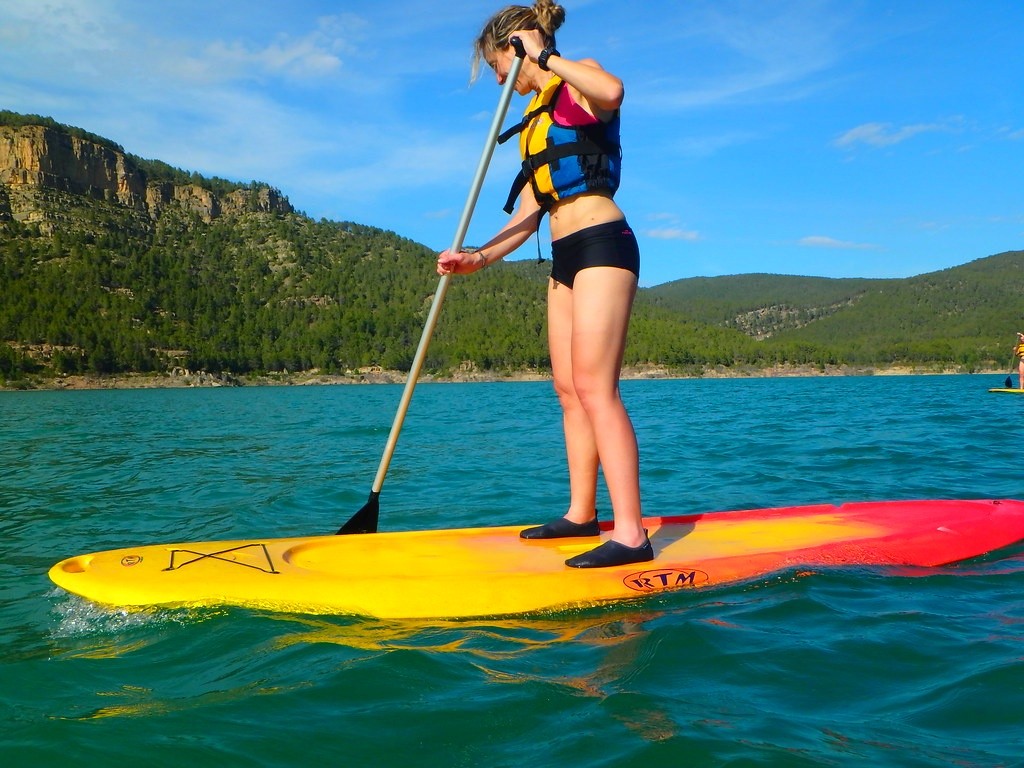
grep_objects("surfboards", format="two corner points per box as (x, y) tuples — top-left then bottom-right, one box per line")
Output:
(40, 492), (1024, 626)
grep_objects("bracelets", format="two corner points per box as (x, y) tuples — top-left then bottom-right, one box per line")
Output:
(474, 250), (486, 268)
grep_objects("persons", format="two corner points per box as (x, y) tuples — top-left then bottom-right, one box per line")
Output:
(1012, 332), (1024, 391)
(437, 1), (653, 568)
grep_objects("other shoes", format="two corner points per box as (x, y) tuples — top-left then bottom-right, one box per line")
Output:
(520, 509), (600, 539)
(565, 528), (653, 568)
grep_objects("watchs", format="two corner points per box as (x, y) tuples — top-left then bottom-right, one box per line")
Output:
(537, 47), (560, 71)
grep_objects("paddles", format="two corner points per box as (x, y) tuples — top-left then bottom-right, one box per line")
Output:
(333, 33), (528, 537)
